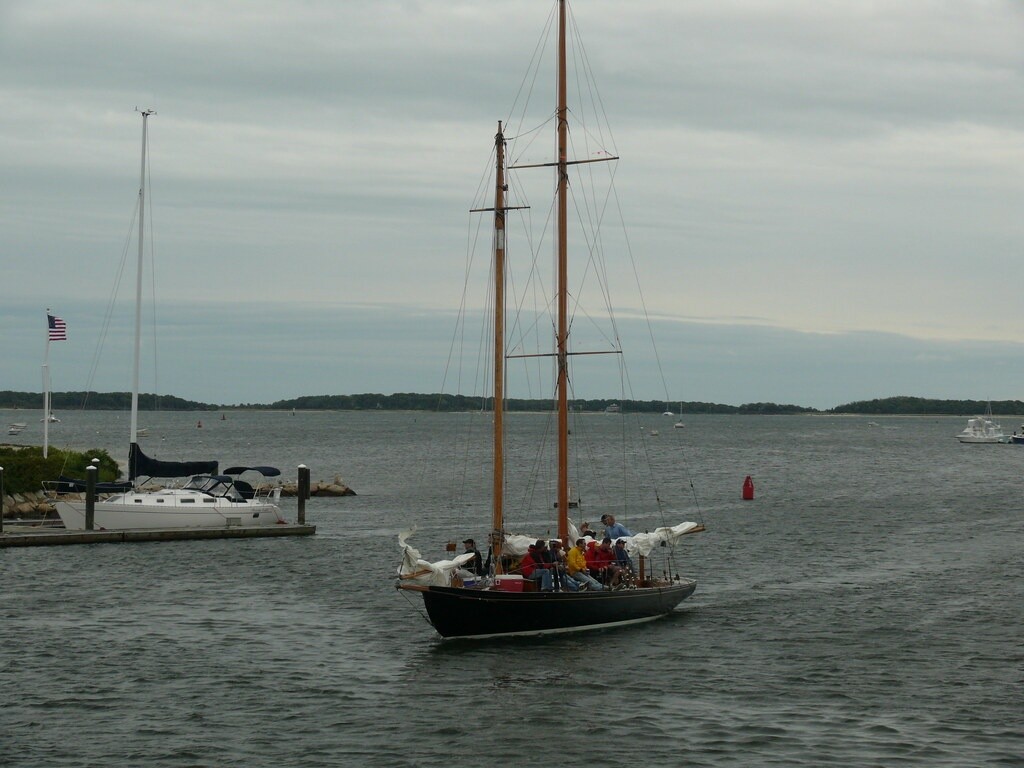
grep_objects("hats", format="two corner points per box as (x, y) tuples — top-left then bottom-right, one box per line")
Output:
(463, 539), (476, 544)
(617, 539), (627, 544)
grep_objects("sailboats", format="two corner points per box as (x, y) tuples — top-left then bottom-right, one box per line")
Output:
(383, 0), (711, 638)
(674, 400), (685, 429)
(662, 394), (673, 415)
(42, 106), (291, 530)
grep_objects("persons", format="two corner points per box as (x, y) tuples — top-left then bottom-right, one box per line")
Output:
(461, 539), (481, 574)
(482, 534), (492, 576)
(585, 537), (637, 588)
(579, 524), (596, 539)
(605, 515), (632, 538)
(520, 540), (553, 591)
(600, 514), (624, 538)
(549, 541), (586, 591)
(567, 539), (623, 591)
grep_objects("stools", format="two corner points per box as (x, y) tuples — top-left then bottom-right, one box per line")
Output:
(524, 578), (540, 592)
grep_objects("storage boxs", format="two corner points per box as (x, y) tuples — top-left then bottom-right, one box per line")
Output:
(462, 576), (482, 586)
(494, 575), (524, 592)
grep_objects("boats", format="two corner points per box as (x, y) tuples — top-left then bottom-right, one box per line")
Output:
(41, 416), (61, 423)
(10, 422), (27, 429)
(8, 429), (20, 436)
(1012, 425), (1024, 443)
(954, 395), (1011, 443)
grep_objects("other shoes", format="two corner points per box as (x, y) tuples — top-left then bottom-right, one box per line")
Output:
(579, 580), (589, 589)
(579, 587), (587, 593)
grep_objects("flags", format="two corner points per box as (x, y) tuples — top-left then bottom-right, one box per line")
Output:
(48, 315), (66, 341)
